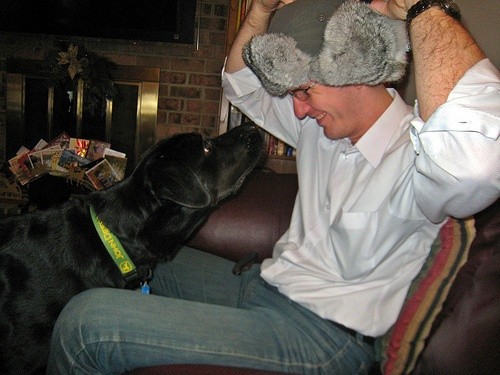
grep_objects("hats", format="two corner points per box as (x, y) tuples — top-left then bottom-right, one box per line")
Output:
(241, 0), (412, 98)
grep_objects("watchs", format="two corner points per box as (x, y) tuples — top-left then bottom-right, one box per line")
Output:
(406, 0), (461, 23)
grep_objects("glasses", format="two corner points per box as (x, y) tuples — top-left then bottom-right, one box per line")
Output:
(286, 81), (314, 102)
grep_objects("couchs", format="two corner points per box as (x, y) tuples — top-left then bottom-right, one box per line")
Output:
(126, 173), (500, 375)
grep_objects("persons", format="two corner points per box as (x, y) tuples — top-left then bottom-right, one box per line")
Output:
(47, 0), (500, 375)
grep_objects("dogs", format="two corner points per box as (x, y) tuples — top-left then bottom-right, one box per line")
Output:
(0, 120), (265, 375)
